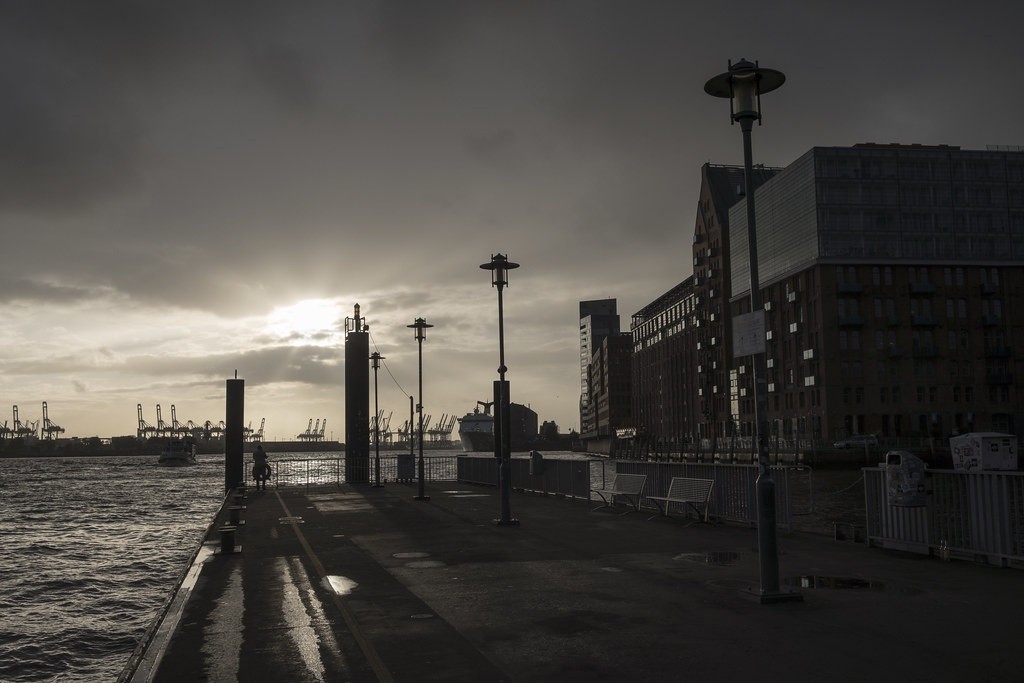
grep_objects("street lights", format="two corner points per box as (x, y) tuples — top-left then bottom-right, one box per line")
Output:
(407, 316), (434, 501)
(369, 353), (387, 488)
(479, 252), (523, 529)
(704, 56), (803, 604)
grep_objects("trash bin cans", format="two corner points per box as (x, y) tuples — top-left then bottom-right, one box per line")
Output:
(885, 450), (933, 508)
(396, 453), (416, 482)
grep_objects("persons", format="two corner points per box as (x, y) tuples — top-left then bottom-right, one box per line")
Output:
(253, 445), (268, 490)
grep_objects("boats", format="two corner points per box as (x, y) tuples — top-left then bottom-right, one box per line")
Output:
(159, 440), (200, 467)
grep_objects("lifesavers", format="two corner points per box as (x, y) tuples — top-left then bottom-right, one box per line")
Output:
(252, 463), (271, 480)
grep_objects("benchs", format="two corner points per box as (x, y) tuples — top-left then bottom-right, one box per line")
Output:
(593, 473), (647, 516)
(646, 477), (715, 529)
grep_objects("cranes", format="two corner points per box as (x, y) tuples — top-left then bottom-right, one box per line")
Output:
(135, 402), (267, 443)
(296, 409), (459, 444)
(0, 400), (66, 442)
